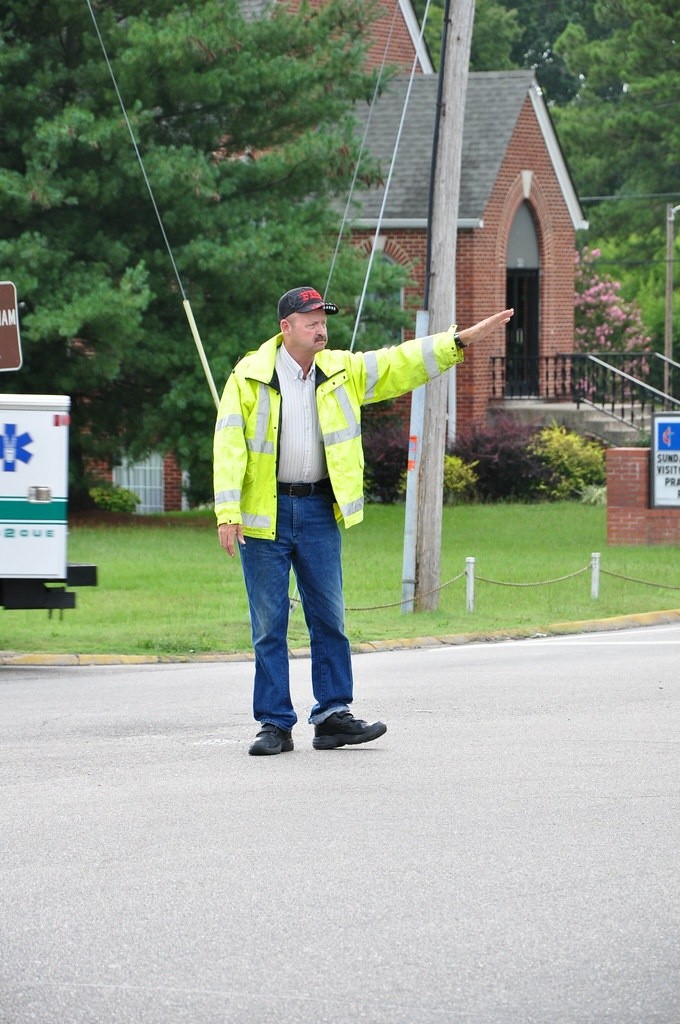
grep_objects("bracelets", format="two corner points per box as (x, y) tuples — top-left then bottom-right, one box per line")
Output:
(454, 332), (469, 349)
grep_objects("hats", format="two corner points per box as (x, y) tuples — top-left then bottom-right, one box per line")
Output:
(277, 287), (339, 322)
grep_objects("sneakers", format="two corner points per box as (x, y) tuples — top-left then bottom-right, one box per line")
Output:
(249, 723), (294, 756)
(312, 711), (387, 750)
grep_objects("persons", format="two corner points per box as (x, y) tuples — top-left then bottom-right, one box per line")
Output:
(212, 286), (515, 757)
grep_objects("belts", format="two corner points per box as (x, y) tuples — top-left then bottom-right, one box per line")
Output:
(278, 478), (331, 498)
